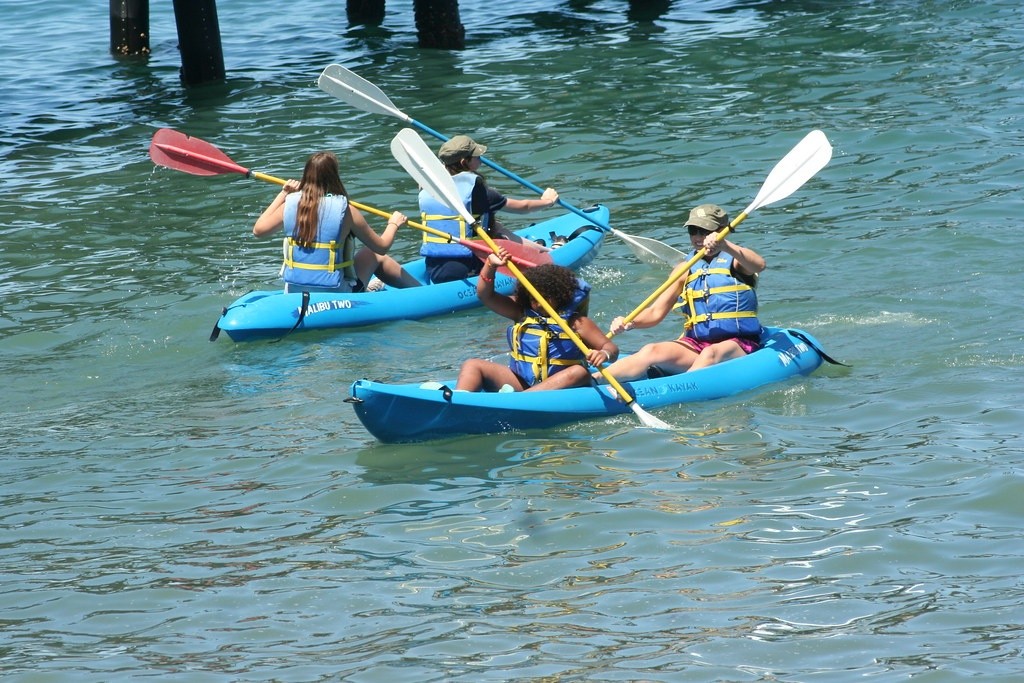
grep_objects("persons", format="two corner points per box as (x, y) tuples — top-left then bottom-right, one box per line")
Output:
(418, 135), (568, 284)
(253, 151), (424, 294)
(420, 246), (619, 392)
(592, 204), (765, 385)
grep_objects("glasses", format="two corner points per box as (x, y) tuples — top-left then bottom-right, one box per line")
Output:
(687, 227), (710, 236)
(470, 156), (480, 160)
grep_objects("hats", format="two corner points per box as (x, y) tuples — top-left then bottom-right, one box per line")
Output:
(438, 135), (487, 165)
(683, 203), (729, 231)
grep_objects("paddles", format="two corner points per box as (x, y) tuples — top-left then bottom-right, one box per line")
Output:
(390, 127), (671, 427)
(317, 65), (689, 268)
(603, 130), (835, 333)
(150, 131), (553, 276)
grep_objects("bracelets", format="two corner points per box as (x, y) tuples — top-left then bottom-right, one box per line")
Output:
(487, 258), (497, 271)
(388, 222), (399, 230)
(480, 271), (495, 282)
(281, 192), (286, 197)
(545, 199), (554, 207)
(625, 321), (635, 331)
(601, 350), (611, 362)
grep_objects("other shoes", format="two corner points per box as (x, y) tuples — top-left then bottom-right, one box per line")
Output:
(500, 384), (517, 393)
(365, 277), (384, 294)
(533, 239), (546, 247)
(552, 236), (567, 250)
(421, 381), (470, 393)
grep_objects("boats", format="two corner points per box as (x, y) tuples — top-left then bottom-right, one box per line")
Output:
(350, 327), (825, 440)
(216, 206), (612, 342)
